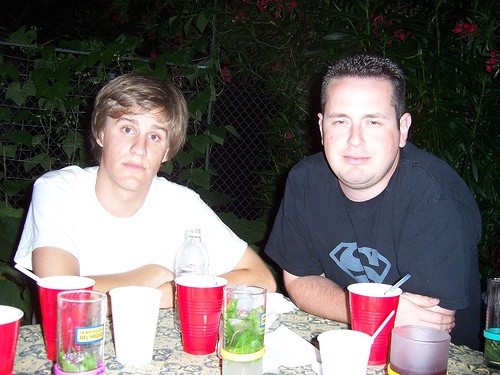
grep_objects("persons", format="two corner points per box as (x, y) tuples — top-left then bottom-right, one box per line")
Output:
(264, 54), (486, 354)
(13, 69), (277, 319)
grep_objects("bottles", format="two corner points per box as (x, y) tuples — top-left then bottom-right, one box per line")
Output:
(174, 227), (209, 332)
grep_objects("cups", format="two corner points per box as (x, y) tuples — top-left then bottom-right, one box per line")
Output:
(109, 286), (162, 365)
(36, 276), (95, 361)
(0, 304), (24, 375)
(174, 275), (228, 355)
(317, 330), (372, 375)
(222, 284), (267, 354)
(347, 283), (402, 366)
(483, 278), (500, 369)
(390, 325), (451, 375)
(57, 290), (107, 372)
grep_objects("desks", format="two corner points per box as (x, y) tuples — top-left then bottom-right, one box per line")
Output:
(12, 294), (500, 375)
